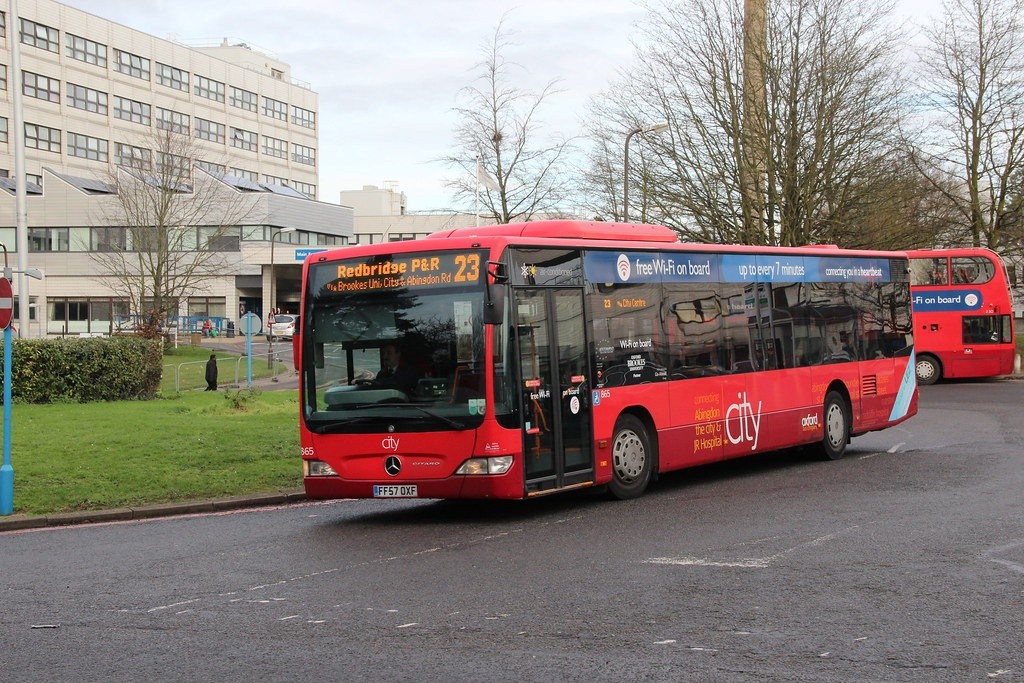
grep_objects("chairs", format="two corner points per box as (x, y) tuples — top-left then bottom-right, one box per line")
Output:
(969, 319), (983, 343)
(450, 364), (504, 406)
(661, 333), (749, 378)
(915, 266), (971, 285)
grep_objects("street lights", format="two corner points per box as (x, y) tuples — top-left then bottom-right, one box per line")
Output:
(624, 122), (668, 222)
(268, 227), (296, 370)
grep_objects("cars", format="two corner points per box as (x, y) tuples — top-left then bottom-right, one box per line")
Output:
(266, 314), (299, 343)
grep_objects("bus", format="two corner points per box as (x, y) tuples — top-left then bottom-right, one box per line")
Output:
(899, 246), (1016, 386)
(291, 219), (918, 502)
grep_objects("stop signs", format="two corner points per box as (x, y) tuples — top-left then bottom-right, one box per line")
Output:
(0, 278), (14, 331)
